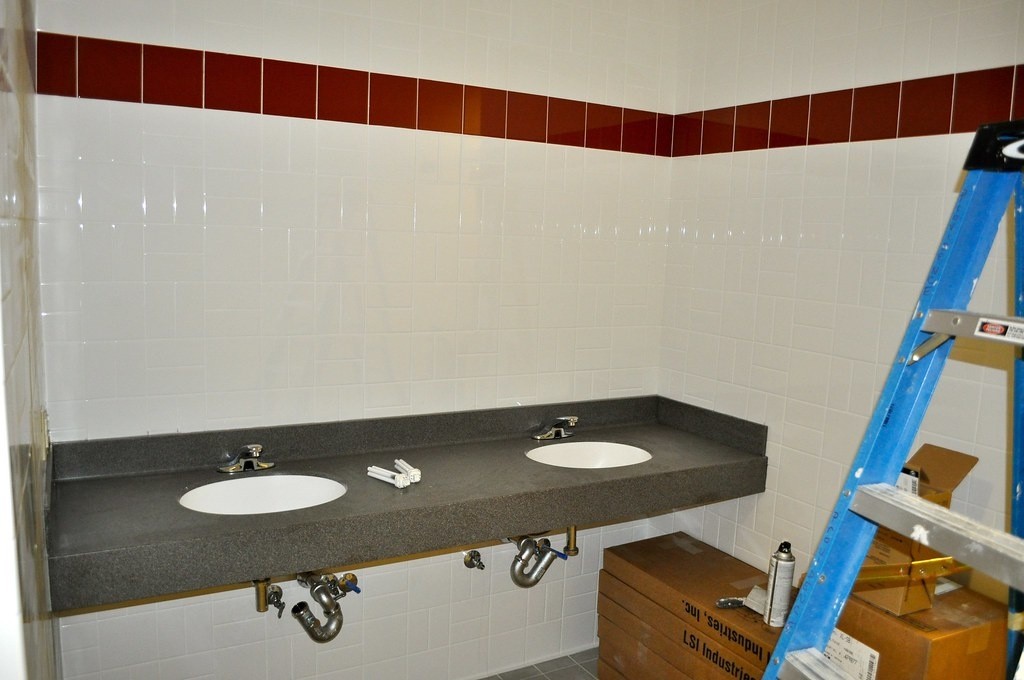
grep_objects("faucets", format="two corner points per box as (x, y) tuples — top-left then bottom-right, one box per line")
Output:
(219, 445), (276, 474)
(533, 416), (578, 440)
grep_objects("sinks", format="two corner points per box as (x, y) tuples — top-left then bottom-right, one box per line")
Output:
(526, 441), (654, 468)
(179, 471), (348, 515)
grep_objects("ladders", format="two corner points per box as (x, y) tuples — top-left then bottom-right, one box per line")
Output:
(763, 118), (1024, 680)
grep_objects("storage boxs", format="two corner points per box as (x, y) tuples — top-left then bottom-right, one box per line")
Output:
(800, 573), (1007, 680)
(852, 443), (979, 616)
(597, 530), (798, 680)
